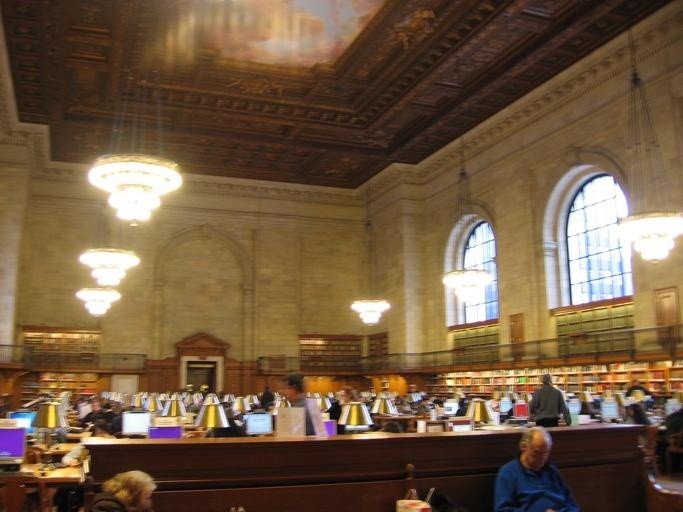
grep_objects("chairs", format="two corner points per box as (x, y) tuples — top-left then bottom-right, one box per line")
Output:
(665, 432), (683, 478)
(639, 425), (661, 480)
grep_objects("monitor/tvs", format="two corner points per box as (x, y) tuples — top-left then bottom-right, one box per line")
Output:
(148, 425), (183, 439)
(246, 412), (273, 435)
(321, 420), (336, 435)
(121, 411), (152, 438)
(0, 427), (26, 460)
(600, 401), (619, 423)
(443, 401), (460, 417)
(500, 401), (513, 416)
(666, 403), (683, 416)
(568, 402), (582, 415)
(9, 412), (39, 436)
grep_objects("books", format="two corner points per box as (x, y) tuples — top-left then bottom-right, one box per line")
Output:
(427, 358), (682, 409)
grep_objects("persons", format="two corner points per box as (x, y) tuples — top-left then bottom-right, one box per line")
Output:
(654, 402), (682, 471)
(107, 407), (125, 434)
(492, 425), (583, 511)
(454, 401), (466, 416)
(323, 397), (341, 420)
(528, 373), (572, 426)
(407, 384), (422, 404)
(622, 402), (651, 446)
(84, 469), (158, 512)
(258, 384), (273, 407)
(96, 402), (115, 420)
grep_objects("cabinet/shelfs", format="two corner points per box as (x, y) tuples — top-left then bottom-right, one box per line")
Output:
(20, 371), (97, 401)
(426, 358), (683, 399)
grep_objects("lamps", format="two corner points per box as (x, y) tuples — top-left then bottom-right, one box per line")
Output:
(80, 223), (142, 288)
(75, 289), (121, 317)
(89, 1), (184, 222)
(351, 224), (392, 325)
(617, 25), (683, 261)
(441, 155), (493, 304)
